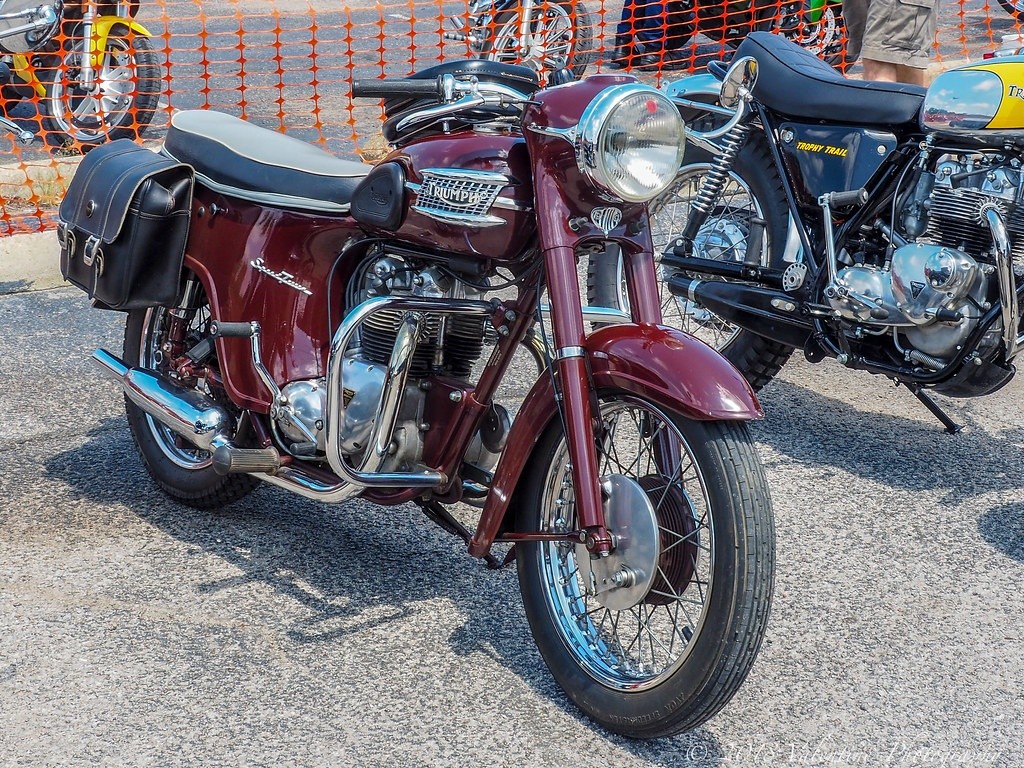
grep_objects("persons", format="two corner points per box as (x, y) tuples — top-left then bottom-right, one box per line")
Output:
(858, 0), (938, 89)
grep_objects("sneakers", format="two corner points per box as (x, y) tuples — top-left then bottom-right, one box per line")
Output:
(639, 44), (687, 70)
(610, 36), (641, 66)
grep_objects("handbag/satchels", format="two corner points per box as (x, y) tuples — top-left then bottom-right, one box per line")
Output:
(56, 139), (195, 311)
(383, 59), (540, 146)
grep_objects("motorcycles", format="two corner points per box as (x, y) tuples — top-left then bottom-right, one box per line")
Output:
(0, 0), (162, 154)
(589, 31), (1024, 430)
(58, 57), (776, 740)
(444, 0), (592, 88)
(634, 0), (862, 77)
